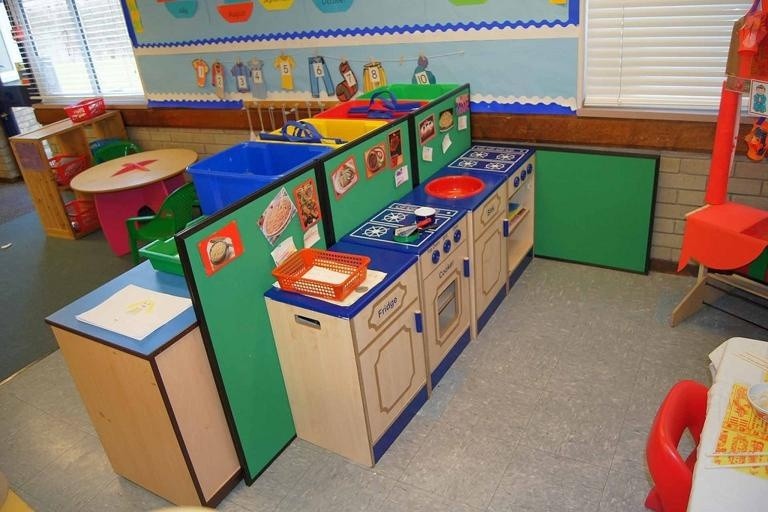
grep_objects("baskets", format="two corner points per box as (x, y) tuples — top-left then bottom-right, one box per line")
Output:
(89, 136), (120, 164)
(64, 199), (98, 233)
(63, 97), (106, 122)
(47, 153), (87, 185)
(271, 247), (371, 301)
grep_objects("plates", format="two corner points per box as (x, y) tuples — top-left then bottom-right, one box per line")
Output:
(334, 163), (358, 194)
(747, 383), (768, 415)
(263, 198), (292, 237)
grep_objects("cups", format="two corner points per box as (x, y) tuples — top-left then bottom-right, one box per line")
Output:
(414, 206), (437, 227)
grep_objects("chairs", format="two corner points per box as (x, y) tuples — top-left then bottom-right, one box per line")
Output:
(91, 140), (143, 166)
(125, 182), (198, 266)
(642, 379), (710, 511)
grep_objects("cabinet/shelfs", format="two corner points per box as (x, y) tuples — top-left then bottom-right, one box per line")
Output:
(7, 110), (129, 241)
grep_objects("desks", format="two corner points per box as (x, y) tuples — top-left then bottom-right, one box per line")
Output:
(687, 338), (768, 511)
(69, 148), (197, 257)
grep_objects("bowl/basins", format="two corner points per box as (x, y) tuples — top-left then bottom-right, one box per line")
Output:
(209, 240), (227, 265)
